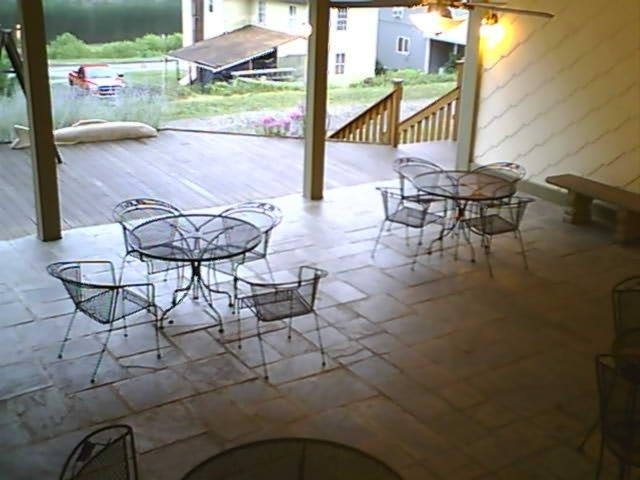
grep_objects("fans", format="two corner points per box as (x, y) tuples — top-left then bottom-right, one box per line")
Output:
(402, 0), (556, 60)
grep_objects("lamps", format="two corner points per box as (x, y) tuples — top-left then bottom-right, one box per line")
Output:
(407, 6), (512, 55)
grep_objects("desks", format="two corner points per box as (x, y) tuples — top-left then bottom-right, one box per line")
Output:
(413, 171), (514, 261)
(611, 325), (639, 366)
(178, 436), (404, 480)
(128, 212), (264, 332)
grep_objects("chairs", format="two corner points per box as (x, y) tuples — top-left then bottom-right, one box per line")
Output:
(595, 353), (640, 480)
(189, 202), (284, 314)
(392, 155), (457, 248)
(371, 184), (446, 269)
(236, 264), (331, 384)
(451, 162), (526, 245)
(112, 197), (200, 326)
(454, 197), (534, 275)
(46, 260), (162, 386)
(59, 424), (140, 480)
(576, 274), (639, 450)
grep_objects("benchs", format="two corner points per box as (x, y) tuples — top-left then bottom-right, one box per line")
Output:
(544, 172), (639, 244)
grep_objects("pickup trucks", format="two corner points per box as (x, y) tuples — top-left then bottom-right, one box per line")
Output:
(66, 61), (129, 99)
